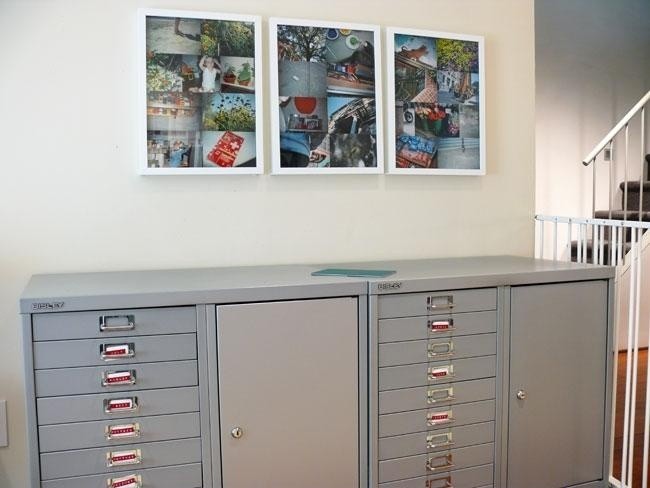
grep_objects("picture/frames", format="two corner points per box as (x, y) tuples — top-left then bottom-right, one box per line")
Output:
(389, 26), (485, 176)
(269, 14), (384, 174)
(136, 10), (266, 176)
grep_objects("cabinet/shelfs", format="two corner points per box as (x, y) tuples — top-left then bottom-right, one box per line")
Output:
(20, 253), (613, 487)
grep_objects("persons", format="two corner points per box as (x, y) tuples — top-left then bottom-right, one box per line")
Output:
(198, 55), (221, 88)
(280, 133), (330, 167)
(148, 139), (193, 168)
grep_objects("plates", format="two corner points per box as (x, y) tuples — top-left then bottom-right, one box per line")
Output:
(340, 29), (351, 36)
(345, 35), (361, 50)
(326, 28), (339, 41)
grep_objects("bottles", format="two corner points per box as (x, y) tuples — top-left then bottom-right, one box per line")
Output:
(288, 113), (302, 130)
